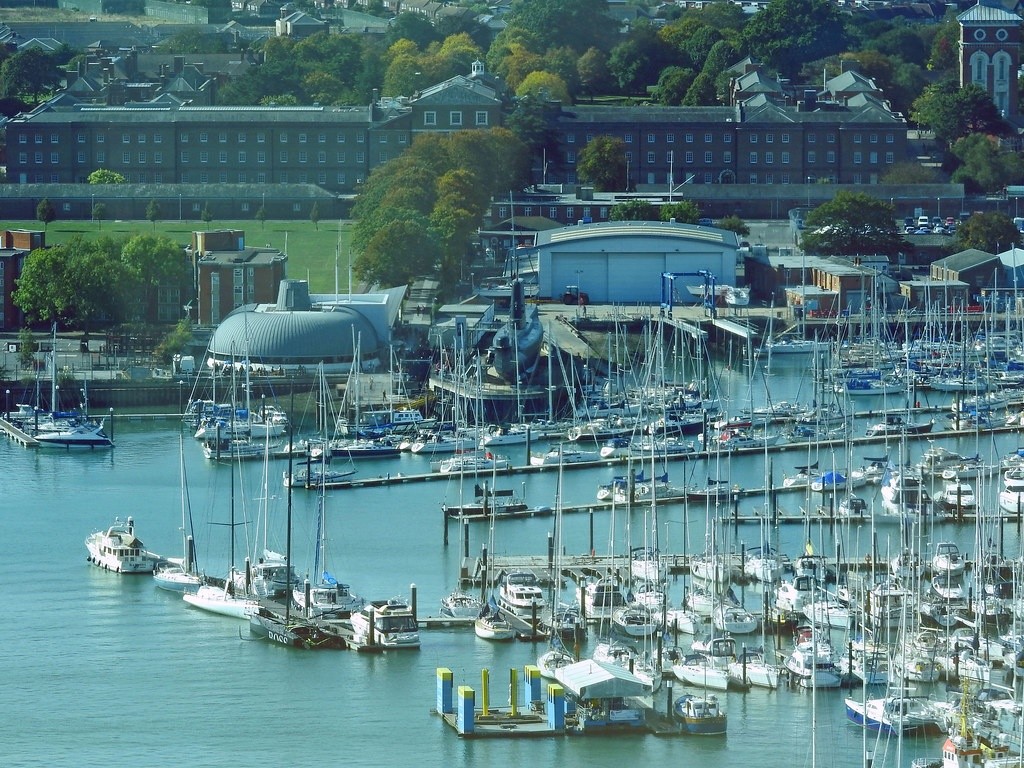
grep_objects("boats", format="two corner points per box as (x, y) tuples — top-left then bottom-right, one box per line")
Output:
(85, 519), (155, 573)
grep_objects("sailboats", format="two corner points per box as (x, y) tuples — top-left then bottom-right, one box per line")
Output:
(2, 321), (117, 448)
(152, 253), (1024, 761)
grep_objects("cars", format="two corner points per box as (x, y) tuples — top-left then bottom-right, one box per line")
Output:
(901, 216), (959, 235)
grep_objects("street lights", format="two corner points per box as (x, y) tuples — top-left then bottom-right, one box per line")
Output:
(807, 176), (811, 209)
(890, 197), (894, 222)
(1015, 197), (1019, 219)
(937, 197), (941, 218)
